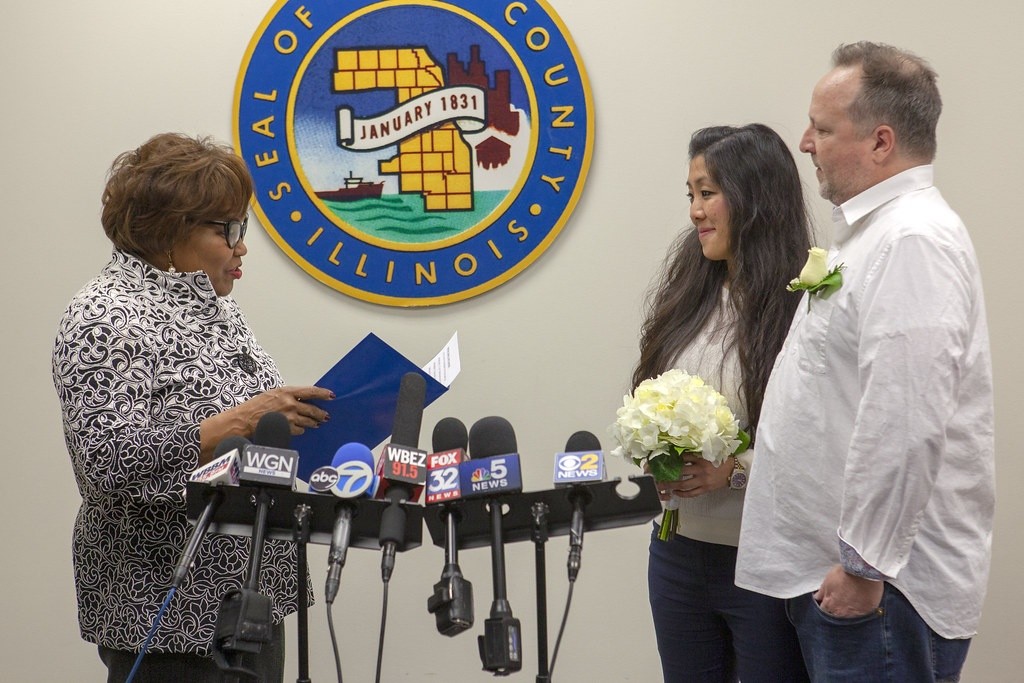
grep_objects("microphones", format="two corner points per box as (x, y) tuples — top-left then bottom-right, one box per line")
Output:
(171, 368), (600, 676)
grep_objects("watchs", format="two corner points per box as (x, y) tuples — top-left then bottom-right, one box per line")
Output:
(727, 455), (748, 495)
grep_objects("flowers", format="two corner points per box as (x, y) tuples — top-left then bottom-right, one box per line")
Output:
(611, 369), (755, 543)
(784, 244), (845, 316)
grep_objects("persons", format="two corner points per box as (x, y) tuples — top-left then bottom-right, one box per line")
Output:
(733, 39), (999, 683)
(629, 123), (826, 683)
(51, 130), (338, 682)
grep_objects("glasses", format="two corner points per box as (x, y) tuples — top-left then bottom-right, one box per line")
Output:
(186, 213), (249, 249)
